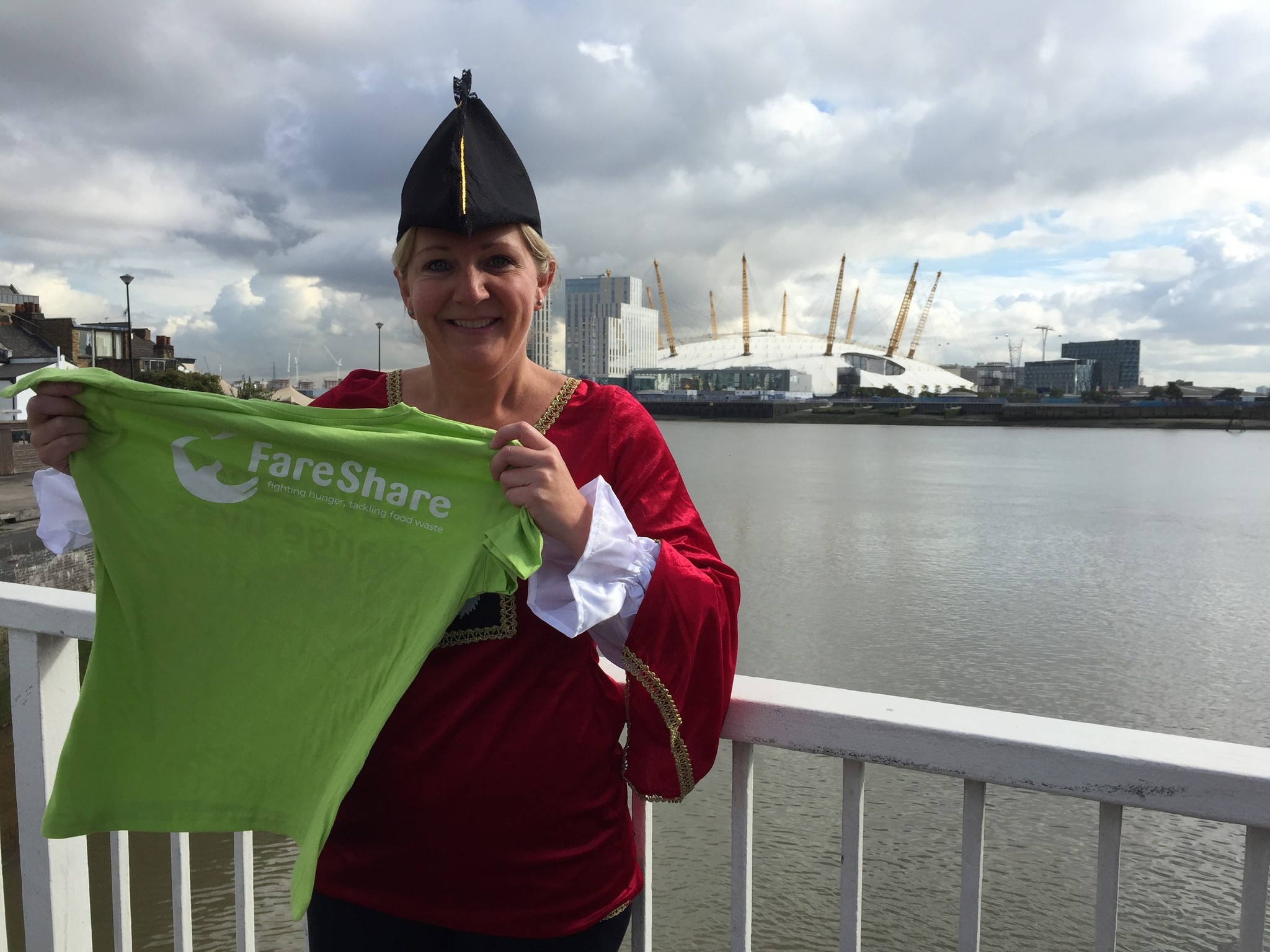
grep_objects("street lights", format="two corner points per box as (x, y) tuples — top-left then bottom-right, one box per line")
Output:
(120, 274), (135, 380)
(375, 321), (384, 371)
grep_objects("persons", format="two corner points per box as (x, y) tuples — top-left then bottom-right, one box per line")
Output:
(27, 67), (744, 952)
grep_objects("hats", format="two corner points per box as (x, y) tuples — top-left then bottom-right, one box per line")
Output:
(397, 67), (545, 242)
